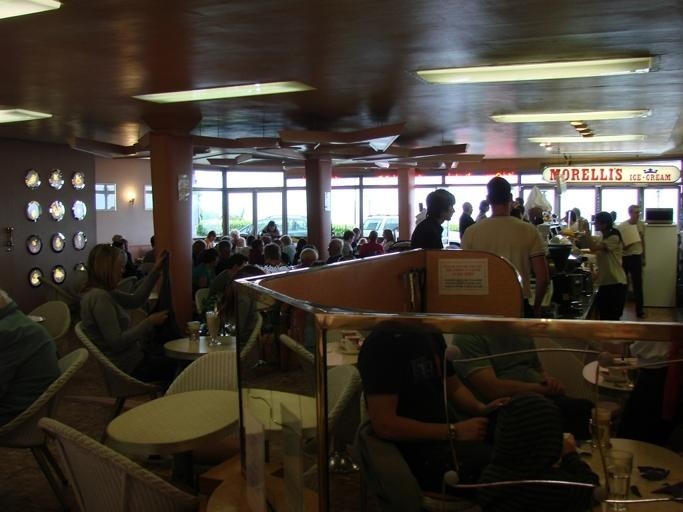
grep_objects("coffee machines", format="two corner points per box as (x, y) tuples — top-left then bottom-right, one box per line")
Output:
(547, 244), (587, 315)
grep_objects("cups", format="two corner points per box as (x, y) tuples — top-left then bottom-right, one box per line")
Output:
(187, 323), (200, 343)
(607, 366), (623, 376)
(591, 407), (611, 448)
(206, 312), (221, 346)
(602, 448), (634, 499)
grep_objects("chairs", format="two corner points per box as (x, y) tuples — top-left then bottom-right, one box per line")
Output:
(241, 311), (682, 511)
(2, 275), (240, 510)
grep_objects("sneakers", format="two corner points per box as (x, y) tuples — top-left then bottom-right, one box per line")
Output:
(636, 310), (648, 319)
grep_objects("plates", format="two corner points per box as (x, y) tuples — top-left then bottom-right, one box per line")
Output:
(600, 370), (626, 382)
